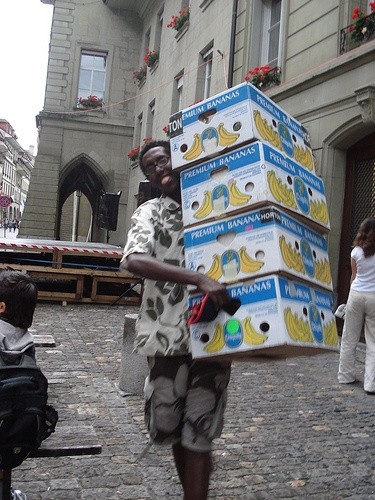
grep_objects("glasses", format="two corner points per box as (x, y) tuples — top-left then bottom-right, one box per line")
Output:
(144, 156), (170, 175)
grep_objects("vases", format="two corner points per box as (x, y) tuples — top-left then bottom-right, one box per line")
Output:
(175, 23), (188, 38)
(130, 161), (137, 166)
(137, 76), (146, 86)
(149, 59), (158, 72)
(79, 104), (102, 110)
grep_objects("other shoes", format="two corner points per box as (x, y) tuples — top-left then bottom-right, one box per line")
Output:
(366, 391), (375, 395)
(341, 379), (361, 384)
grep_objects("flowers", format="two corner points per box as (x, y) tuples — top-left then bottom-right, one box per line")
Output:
(245, 65), (282, 90)
(141, 137), (152, 149)
(163, 125), (169, 137)
(348, 1), (375, 41)
(77, 96), (104, 108)
(132, 66), (147, 84)
(167, 7), (190, 30)
(128, 145), (139, 162)
(144, 47), (159, 67)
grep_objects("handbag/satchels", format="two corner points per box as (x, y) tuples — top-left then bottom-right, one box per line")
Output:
(335, 304), (346, 319)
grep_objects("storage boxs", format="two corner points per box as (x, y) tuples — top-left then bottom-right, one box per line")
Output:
(169, 82), (316, 175)
(189, 275), (340, 361)
(180, 141), (331, 235)
(182, 206), (334, 293)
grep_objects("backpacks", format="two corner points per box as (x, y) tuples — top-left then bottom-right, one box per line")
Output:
(0, 354), (58, 471)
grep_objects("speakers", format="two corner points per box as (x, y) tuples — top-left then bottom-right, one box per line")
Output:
(97, 193), (120, 231)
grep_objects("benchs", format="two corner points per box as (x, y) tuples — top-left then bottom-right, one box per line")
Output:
(1, 425), (102, 500)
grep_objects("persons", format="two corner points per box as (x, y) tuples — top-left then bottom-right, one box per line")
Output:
(0, 217), (18, 233)
(120, 141), (233, 500)
(338, 218), (375, 396)
(0, 270), (35, 461)
